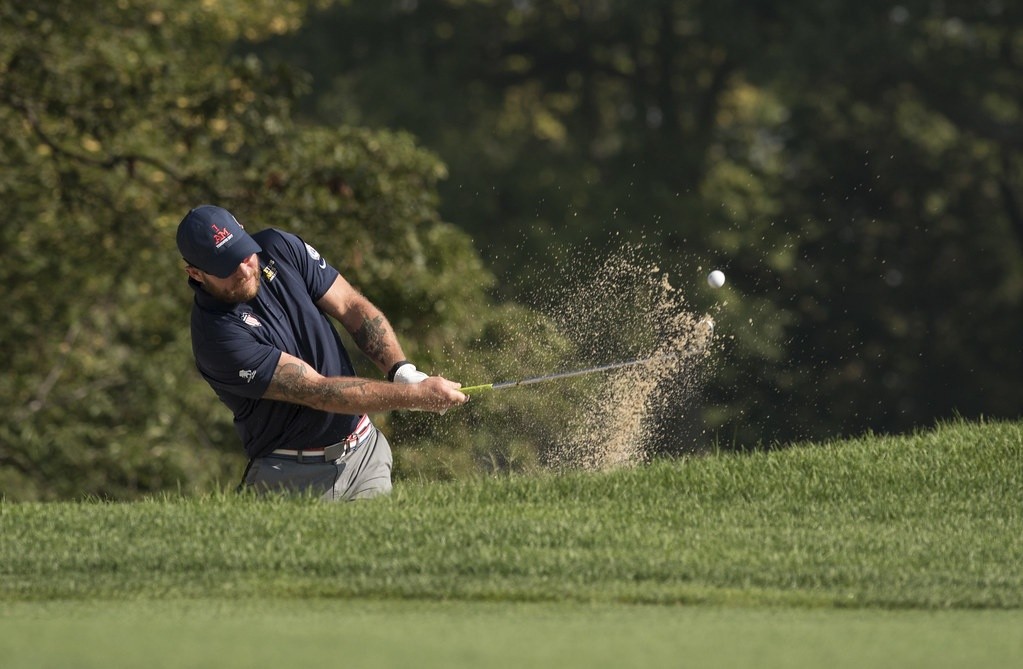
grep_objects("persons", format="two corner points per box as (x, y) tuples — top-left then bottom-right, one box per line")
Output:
(176, 205), (469, 500)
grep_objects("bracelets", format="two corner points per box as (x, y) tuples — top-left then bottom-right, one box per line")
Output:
(387, 361), (415, 382)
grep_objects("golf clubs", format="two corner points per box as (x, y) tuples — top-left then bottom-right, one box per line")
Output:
(454, 320), (713, 397)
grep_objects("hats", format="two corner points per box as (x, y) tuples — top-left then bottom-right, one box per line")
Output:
(176, 205), (262, 279)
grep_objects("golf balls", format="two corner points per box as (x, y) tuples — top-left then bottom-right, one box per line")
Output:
(707, 269), (726, 290)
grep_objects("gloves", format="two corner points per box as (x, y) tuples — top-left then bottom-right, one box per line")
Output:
(387, 360), (447, 416)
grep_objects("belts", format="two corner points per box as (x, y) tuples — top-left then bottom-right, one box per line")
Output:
(267, 413), (372, 462)
